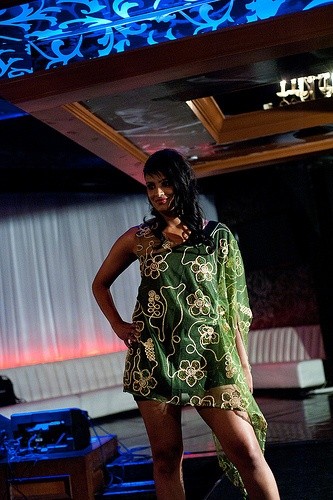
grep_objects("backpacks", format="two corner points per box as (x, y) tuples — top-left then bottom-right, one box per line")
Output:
(0, 375), (27, 408)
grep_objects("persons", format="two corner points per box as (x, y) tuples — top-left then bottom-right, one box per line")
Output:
(91, 148), (282, 500)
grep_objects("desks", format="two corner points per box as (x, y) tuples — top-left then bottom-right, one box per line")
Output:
(0, 434), (117, 500)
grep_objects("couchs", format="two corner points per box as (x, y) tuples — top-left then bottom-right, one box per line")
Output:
(0, 324), (326, 420)
